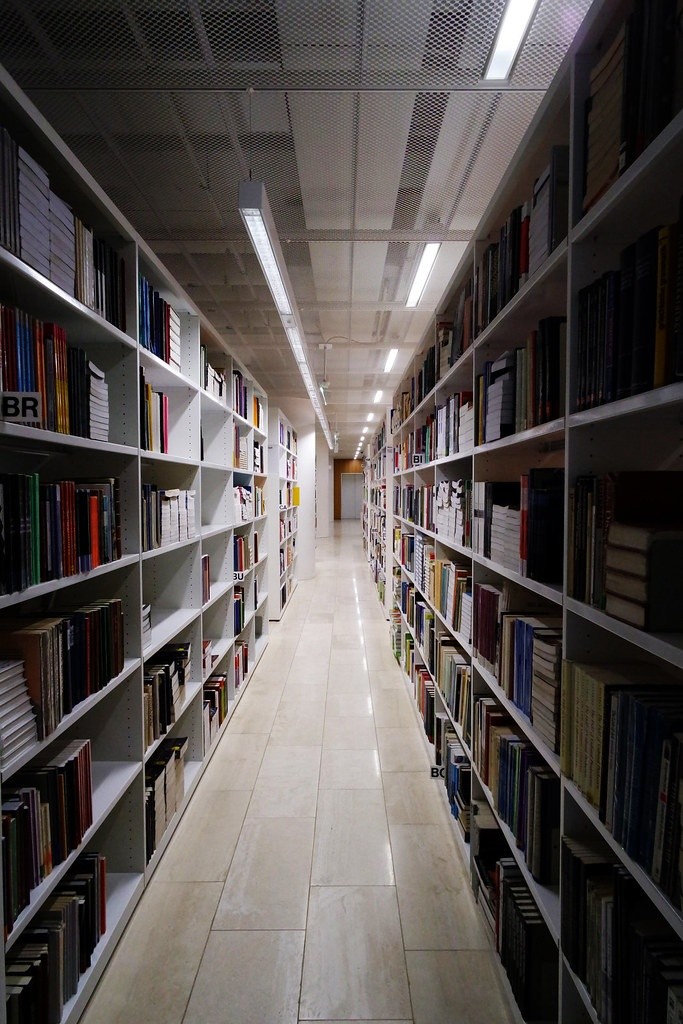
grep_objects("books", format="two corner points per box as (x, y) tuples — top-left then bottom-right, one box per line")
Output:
(2, 142), (303, 1024)
(363, 0), (683, 1024)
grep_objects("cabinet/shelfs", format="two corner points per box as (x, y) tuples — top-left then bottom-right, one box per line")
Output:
(0, 68), (269, 1024)
(268, 407), (299, 622)
(362, 407), (393, 619)
(393, 0), (683, 1024)
